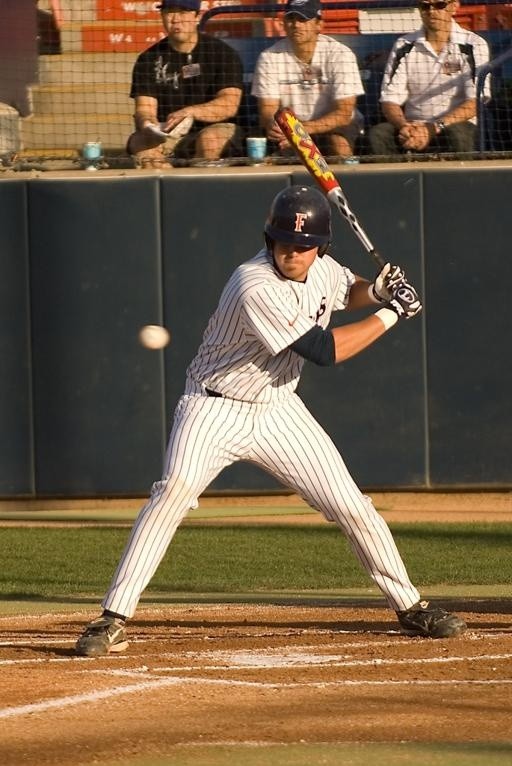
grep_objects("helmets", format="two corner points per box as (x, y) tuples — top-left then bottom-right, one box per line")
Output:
(263, 183), (333, 261)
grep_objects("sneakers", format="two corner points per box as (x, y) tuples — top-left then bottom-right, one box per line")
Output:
(73, 613), (130, 658)
(394, 598), (467, 639)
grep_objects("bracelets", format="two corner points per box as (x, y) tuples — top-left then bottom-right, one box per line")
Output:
(433, 115), (448, 135)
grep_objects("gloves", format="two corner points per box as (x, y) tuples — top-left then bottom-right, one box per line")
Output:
(385, 283), (424, 322)
(372, 261), (407, 303)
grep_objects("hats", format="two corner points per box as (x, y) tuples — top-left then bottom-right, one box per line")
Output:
(283, 0), (321, 20)
(156, 0), (200, 13)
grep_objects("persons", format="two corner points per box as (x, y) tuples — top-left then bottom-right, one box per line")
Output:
(73, 183), (469, 658)
(124, 0), (247, 169)
(361, 0), (493, 156)
(251, 0), (366, 163)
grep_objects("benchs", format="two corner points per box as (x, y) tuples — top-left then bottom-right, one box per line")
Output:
(196, 1), (511, 152)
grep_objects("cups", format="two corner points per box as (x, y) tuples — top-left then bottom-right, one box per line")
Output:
(83, 141), (102, 171)
(246, 136), (268, 162)
(337, 156), (358, 163)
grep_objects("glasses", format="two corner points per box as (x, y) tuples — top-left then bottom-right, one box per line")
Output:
(417, 2), (447, 11)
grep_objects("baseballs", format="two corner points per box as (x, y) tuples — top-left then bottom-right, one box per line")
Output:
(141, 326), (167, 350)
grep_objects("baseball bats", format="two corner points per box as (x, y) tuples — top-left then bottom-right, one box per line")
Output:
(274, 106), (386, 269)
